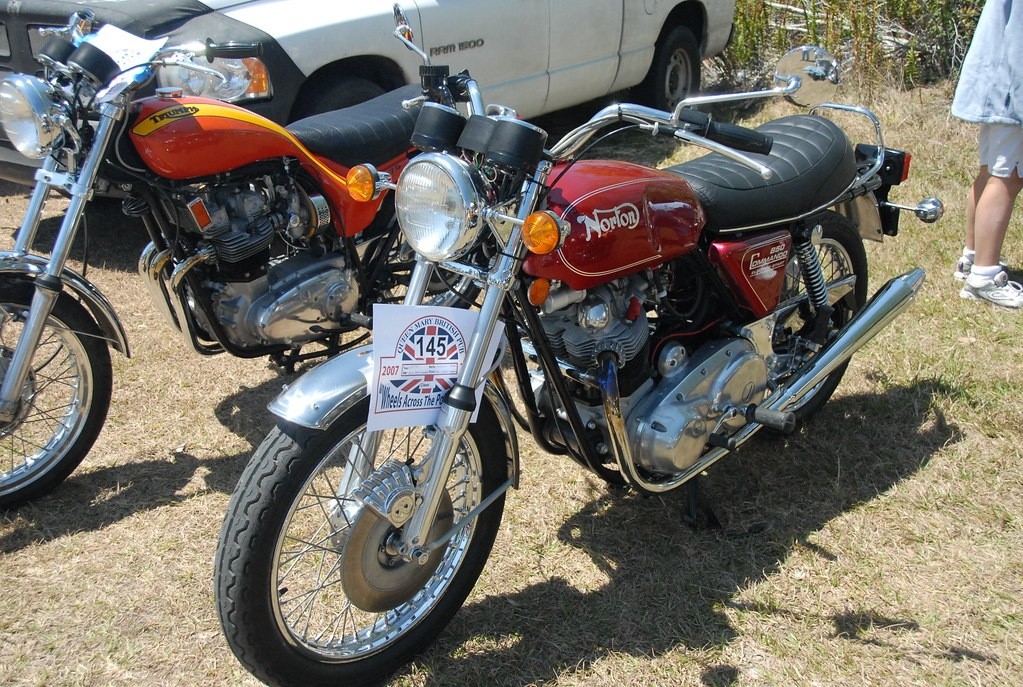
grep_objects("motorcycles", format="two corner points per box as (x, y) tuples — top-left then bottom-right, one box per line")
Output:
(0, 1), (512, 508)
(208, 5), (945, 687)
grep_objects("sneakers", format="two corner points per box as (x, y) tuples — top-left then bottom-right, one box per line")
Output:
(954, 257), (1015, 284)
(959, 271), (1023, 312)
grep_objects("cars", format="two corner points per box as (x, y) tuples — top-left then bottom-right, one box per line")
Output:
(0, 0), (742, 200)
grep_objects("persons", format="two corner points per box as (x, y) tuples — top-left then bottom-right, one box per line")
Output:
(951, 0), (1023, 308)
